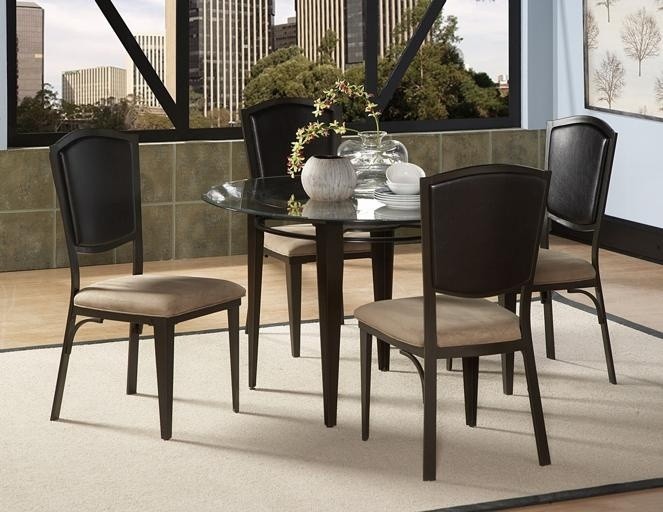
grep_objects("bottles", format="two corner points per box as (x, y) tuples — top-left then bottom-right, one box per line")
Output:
(300, 155), (357, 202)
(302, 199), (357, 221)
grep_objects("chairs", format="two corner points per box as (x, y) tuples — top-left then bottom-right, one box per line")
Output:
(344, 160), (556, 483)
(228, 95), (399, 362)
(439, 110), (623, 399)
(36, 121), (248, 446)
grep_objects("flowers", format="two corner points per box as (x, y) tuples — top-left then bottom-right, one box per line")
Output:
(279, 77), (403, 180)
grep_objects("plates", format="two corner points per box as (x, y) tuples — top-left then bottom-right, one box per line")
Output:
(374, 205), (420, 219)
(374, 189), (420, 211)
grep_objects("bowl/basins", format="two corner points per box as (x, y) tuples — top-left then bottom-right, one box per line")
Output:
(386, 163), (425, 184)
(385, 182), (419, 194)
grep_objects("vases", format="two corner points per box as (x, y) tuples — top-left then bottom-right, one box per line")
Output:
(330, 124), (409, 201)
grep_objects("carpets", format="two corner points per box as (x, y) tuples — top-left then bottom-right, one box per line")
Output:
(0, 291), (663, 510)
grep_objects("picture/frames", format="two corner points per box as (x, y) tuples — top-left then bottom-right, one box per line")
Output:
(580, 0), (663, 124)
